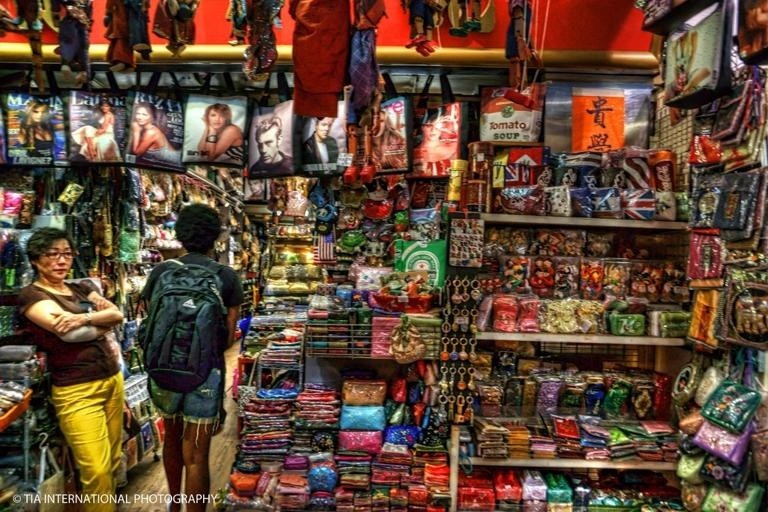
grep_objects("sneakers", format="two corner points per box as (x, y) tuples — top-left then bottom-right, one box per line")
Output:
(167, 496), (172, 512)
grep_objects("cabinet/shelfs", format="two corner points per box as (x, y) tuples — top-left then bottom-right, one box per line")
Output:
(448, 210), (690, 472)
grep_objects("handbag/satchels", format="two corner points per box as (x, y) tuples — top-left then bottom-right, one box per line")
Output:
(7, 89), (467, 178)
(392, 322), (426, 364)
(93, 170), (142, 263)
(664, 0), (735, 109)
(479, 84), (545, 142)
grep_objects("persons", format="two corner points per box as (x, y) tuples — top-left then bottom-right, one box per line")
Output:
(71, 99), (122, 163)
(370, 109), (405, 169)
(28, 99), (66, 162)
(301, 117), (340, 170)
(249, 116), (294, 176)
(195, 103), (246, 167)
(665, 31), (710, 104)
(16, 227), (124, 512)
(413, 107), (458, 177)
(139, 204), (245, 511)
(131, 103), (184, 169)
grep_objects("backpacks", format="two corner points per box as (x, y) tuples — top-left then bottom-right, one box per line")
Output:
(144, 264), (219, 394)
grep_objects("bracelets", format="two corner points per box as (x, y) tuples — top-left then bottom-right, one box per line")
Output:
(84, 312), (91, 326)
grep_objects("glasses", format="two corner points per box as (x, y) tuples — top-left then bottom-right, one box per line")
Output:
(44, 251), (76, 259)
